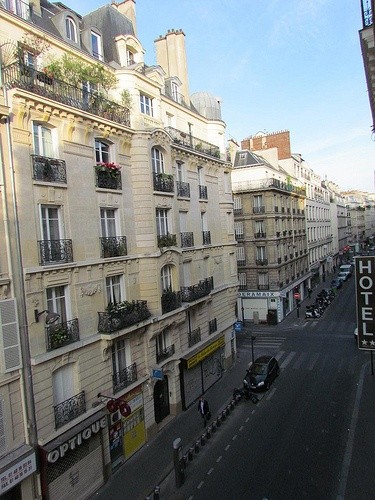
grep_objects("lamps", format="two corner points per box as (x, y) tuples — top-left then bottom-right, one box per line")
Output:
(34, 309), (60, 325)
(288, 244), (296, 249)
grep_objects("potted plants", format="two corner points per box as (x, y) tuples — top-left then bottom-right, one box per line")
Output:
(36, 67), (53, 85)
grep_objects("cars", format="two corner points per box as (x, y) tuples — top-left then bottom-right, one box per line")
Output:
(369, 246), (375, 251)
(361, 250), (370, 256)
(330, 255), (361, 289)
(242, 356), (280, 393)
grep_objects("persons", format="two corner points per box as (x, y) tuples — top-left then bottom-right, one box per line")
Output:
(308, 288), (312, 298)
(198, 396), (209, 428)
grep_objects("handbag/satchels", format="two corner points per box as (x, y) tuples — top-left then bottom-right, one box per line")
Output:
(205, 412), (212, 420)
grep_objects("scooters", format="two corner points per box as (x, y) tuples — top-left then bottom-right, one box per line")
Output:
(306, 289), (335, 319)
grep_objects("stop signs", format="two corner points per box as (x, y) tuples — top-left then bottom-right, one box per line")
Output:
(294, 293), (299, 300)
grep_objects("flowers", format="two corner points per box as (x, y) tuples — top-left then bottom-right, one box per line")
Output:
(95, 161), (122, 174)
